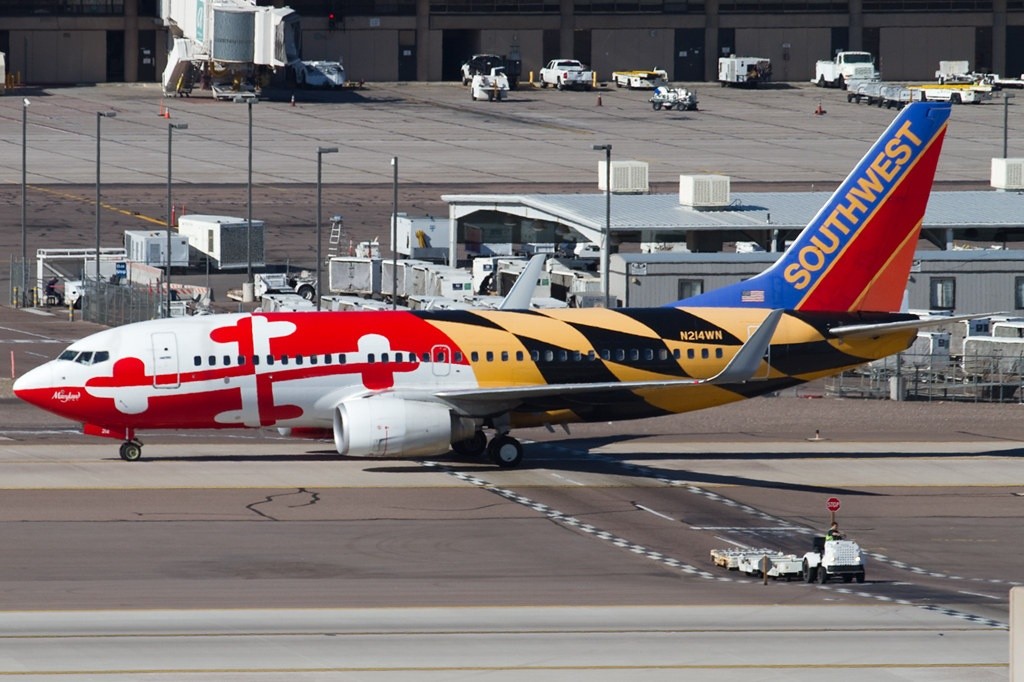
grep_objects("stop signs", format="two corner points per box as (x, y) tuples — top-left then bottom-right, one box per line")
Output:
(826, 497), (841, 512)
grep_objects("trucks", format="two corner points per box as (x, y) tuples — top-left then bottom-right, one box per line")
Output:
(811, 50), (876, 88)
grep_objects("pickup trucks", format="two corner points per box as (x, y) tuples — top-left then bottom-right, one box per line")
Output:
(538, 58), (591, 91)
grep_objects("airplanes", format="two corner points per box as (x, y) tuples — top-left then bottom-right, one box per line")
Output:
(11, 97), (946, 464)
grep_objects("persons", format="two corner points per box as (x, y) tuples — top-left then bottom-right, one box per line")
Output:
(478, 271), (495, 294)
(824, 522), (845, 542)
(47, 277), (63, 303)
